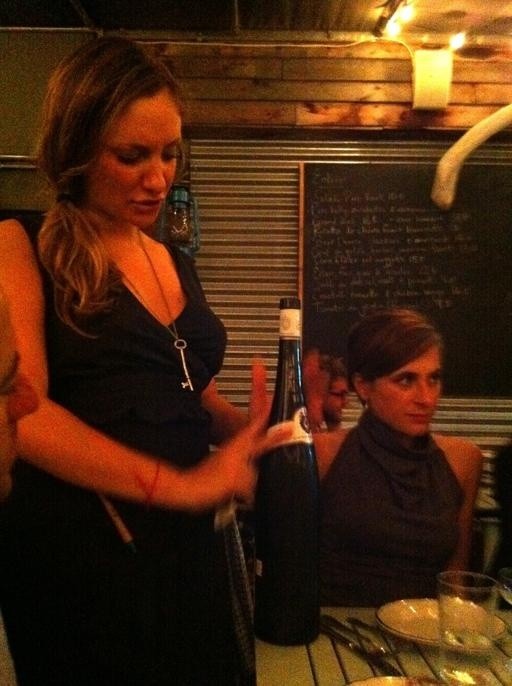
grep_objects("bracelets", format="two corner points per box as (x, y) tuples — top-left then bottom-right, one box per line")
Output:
(140, 458), (160, 507)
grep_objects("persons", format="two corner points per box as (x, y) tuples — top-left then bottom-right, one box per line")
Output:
(314, 309), (484, 608)
(319, 354), (348, 432)
(0, 36), (324, 686)
(0, 289), (39, 686)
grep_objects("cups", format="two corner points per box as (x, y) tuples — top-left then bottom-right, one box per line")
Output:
(436, 571), (503, 685)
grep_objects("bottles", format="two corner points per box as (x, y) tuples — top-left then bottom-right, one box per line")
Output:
(248, 296), (325, 647)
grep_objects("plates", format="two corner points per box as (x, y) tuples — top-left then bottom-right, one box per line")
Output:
(374, 597), (507, 649)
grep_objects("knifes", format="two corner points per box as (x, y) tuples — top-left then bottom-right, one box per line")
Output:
(318, 623), (404, 676)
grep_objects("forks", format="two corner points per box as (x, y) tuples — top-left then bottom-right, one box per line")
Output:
(322, 614), (401, 659)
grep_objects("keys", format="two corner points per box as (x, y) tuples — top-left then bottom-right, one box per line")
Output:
(173, 339), (194, 392)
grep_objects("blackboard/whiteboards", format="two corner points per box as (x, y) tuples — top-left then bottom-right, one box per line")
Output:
(298, 160), (512, 399)
